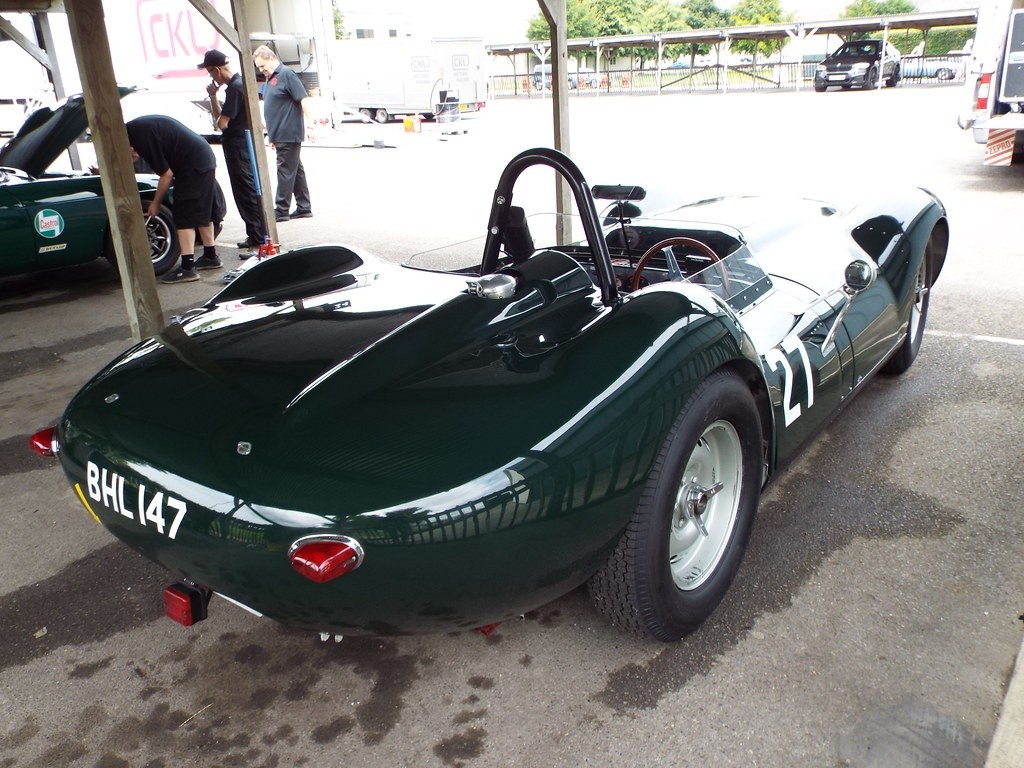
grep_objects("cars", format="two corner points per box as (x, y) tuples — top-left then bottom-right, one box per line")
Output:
(30, 146), (950, 643)
(575, 67), (611, 88)
(0, 87), (182, 279)
(632, 57), (764, 68)
(902, 56), (958, 80)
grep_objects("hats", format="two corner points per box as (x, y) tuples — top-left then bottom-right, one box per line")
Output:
(198, 50), (230, 69)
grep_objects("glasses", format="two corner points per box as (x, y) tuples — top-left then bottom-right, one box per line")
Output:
(206, 67), (219, 77)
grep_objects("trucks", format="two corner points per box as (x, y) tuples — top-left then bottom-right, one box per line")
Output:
(203, 32), (323, 144)
(337, 38), (493, 123)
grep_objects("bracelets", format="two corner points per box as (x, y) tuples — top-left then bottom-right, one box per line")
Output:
(209, 95), (216, 98)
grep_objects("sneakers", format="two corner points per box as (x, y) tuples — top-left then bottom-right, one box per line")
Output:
(274, 210), (312, 221)
(161, 257), (222, 283)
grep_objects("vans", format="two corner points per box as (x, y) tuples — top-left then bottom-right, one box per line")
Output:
(533, 63), (591, 91)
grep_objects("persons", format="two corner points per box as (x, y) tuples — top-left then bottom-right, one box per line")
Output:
(125, 114), (223, 282)
(203, 50), (270, 248)
(253, 45), (313, 222)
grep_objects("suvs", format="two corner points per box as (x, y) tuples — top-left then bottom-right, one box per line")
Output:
(813, 39), (901, 91)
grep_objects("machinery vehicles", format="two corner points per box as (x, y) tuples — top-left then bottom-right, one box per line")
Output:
(956, 1), (1024, 167)
